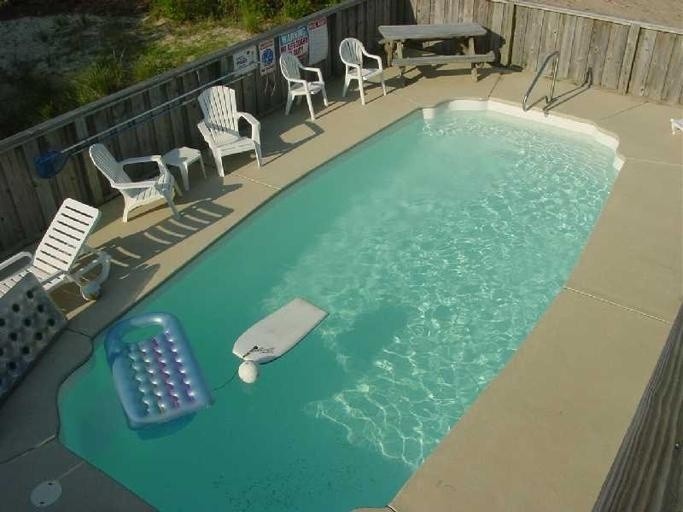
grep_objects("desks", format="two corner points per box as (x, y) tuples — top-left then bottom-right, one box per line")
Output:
(163, 146), (207, 192)
(378, 22), (487, 87)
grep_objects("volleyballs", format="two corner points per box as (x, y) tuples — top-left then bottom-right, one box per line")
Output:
(237, 361), (261, 384)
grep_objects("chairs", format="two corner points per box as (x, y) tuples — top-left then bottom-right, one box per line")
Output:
(0, 196), (111, 316)
(279, 37), (386, 121)
(88, 143), (182, 223)
(196, 84), (262, 176)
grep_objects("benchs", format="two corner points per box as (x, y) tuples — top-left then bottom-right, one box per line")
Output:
(378, 39), (496, 86)
